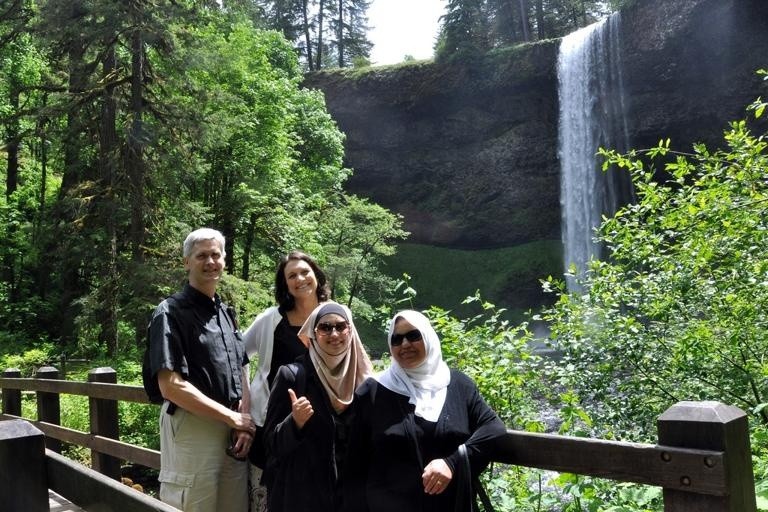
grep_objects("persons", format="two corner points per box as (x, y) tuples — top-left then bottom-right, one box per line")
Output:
(149, 228), (256, 512)
(241, 252), (333, 512)
(264, 301), (373, 512)
(342, 309), (507, 512)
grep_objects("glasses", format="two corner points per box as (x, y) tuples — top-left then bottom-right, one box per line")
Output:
(314, 322), (351, 337)
(391, 329), (420, 346)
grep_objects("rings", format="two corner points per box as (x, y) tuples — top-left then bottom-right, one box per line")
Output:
(437, 480), (442, 486)
